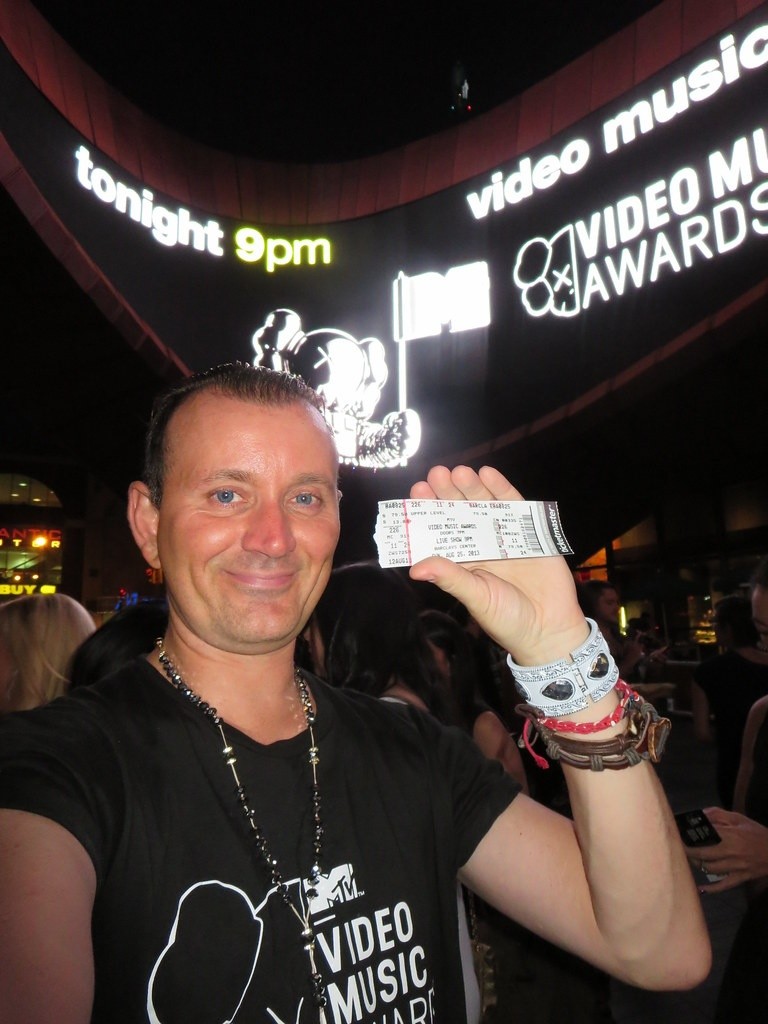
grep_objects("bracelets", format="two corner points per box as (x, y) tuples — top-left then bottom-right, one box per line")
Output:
(516, 697), (672, 770)
(523, 680), (639, 770)
(506, 616), (619, 714)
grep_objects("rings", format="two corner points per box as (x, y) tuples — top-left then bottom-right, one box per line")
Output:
(700, 861), (708, 874)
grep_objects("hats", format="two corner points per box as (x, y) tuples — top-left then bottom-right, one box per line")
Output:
(709, 598), (753, 622)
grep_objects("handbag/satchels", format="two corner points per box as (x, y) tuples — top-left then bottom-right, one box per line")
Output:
(469, 940), (497, 1018)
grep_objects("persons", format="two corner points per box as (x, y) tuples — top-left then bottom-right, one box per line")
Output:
(0, 363), (768, 1024)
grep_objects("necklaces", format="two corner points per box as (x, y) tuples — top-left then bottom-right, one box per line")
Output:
(157, 637), (326, 1007)
